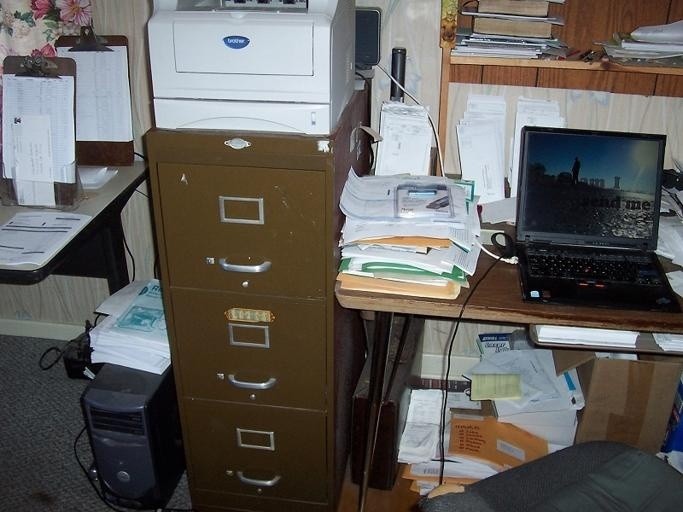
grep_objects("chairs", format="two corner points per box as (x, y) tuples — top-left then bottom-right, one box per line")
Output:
(420, 442), (681, 511)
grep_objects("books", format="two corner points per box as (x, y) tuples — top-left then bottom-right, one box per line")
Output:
(334, 166), (483, 302)
(530, 324), (640, 350)
(86, 275), (173, 375)
(0, 211), (92, 266)
(399, 318), (586, 496)
(450, 0), (682, 68)
(594, 351), (638, 362)
(654, 184), (683, 267)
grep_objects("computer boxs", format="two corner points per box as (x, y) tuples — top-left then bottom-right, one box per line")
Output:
(78, 364), (186, 512)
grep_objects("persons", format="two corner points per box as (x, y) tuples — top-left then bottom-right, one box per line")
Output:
(571, 157), (581, 184)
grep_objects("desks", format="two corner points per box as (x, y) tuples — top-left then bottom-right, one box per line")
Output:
(2, 158), (147, 297)
(335, 200), (682, 491)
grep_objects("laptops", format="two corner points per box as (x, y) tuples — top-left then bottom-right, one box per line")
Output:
(515, 125), (681, 312)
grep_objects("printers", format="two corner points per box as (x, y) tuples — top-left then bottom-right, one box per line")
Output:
(146, 0), (356, 137)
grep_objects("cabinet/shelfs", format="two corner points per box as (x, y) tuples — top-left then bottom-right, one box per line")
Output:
(437, 0), (683, 175)
(142, 88), (371, 511)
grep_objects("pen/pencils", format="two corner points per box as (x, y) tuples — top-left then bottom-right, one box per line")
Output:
(558, 49), (605, 65)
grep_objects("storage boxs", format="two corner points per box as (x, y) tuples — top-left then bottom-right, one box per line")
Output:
(551, 352), (683, 455)
